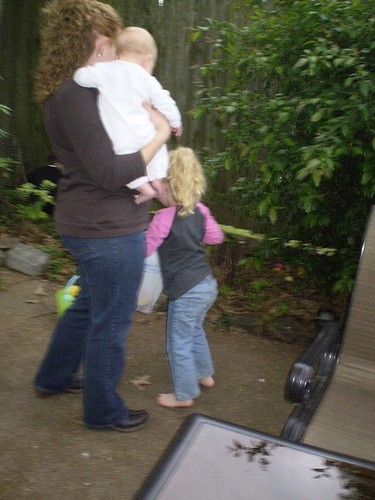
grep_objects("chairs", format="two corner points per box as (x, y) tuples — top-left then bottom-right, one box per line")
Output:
(281, 204), (375, 465)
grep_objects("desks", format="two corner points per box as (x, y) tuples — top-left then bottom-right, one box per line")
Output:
(133, 412), (375, 500)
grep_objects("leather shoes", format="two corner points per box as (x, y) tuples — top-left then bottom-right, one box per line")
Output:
(34, 376), (83, 398)
(111, 409), (150, 433)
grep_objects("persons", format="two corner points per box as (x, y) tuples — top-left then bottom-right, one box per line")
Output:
(33, 1), (172, 432)
(73, 26), (182, 205)
(145, 145), (224, 407)
